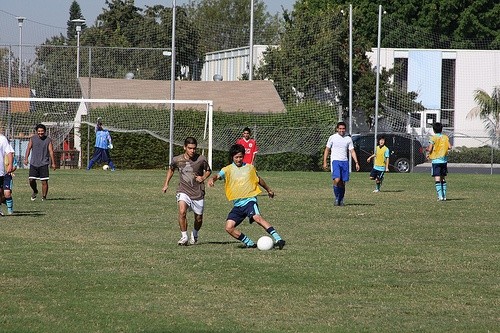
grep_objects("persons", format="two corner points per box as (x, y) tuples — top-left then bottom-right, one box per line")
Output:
(236, 128), (258, 165)
(87, 117), (116, 170)
(25, 124), (57, 201)
(323, 122), (360, 206)
(426, 123), (452, 201)
(162, 138), (212, 245)
(2, 148), (17, 205)
(0, 133), (15, 216)
(367, 136), (390, 193)
(208, 144), (285, 250)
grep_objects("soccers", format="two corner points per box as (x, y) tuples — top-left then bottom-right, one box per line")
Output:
(257, 236), (274, 251)
(103, 165), (109, 171)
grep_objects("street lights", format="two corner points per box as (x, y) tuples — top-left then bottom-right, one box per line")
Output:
(16, 16), (26, 84)
(70, 19), (86, 79)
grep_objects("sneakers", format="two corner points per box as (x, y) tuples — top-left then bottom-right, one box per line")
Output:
(177, 231), (199, 246)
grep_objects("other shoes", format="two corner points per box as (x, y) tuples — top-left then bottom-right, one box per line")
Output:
(275, 239), (286, 250)
(30, 190), (38, 201)
(334, 199), (344, 206)
(437, 196), (447, 201)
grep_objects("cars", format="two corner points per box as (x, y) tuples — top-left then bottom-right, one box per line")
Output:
(347, 132), (425, 174)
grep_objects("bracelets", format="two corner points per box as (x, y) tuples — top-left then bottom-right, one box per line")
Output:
(355, 162), (359, 163)
